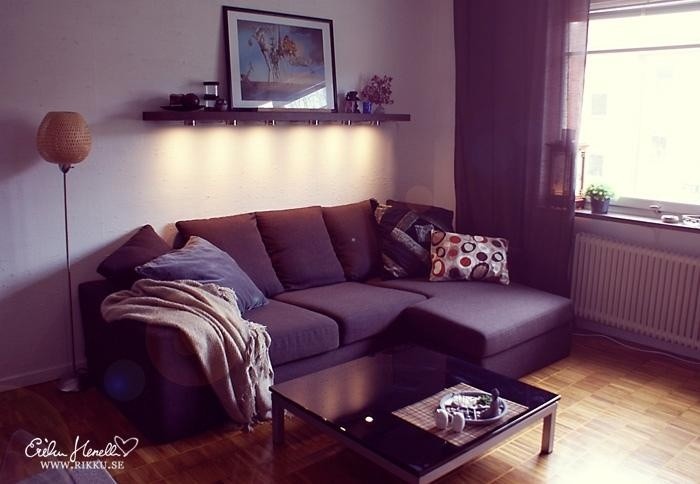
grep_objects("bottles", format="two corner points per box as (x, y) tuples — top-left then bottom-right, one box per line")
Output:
(434, 409), (448, 429)
(452, 411), (466, 432)
(362, 101), (372, 113)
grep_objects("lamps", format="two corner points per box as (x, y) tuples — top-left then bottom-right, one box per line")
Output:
(35, 108), (93, 396)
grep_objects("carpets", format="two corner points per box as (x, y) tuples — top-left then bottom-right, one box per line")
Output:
(18, 459), (117, 484)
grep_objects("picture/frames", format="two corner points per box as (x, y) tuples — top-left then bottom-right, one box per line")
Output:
(223, 2), (338, 115)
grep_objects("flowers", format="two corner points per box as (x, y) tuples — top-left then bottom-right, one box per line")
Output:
(362, 73), (396, 105)
(584, 182), (614, 202)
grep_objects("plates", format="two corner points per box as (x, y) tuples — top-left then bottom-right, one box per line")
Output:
(158, 104), (204, 111)
(440, 391), (507, 424)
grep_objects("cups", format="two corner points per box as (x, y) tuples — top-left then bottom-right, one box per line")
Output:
(169, 94), (184, 104)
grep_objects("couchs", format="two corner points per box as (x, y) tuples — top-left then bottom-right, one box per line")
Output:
(73, 264), (571, 440)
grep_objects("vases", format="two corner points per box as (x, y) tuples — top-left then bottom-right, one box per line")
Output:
(589, 197), (610, 214)
(373, 102), (386, 113)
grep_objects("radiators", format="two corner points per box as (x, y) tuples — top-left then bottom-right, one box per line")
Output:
(570, 229), (700, 351)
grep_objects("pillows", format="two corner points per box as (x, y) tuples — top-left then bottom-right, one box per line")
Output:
(370, 198), (443, 280)
(175, 213), (284, 301)
(96, 224), (175, 291)
(428, 227), (512, 288)
(385, 198), (454, 233)
(321, 197), (379, 282)
(255, 205), (347, 290)
(135, 234), (268, 317)
